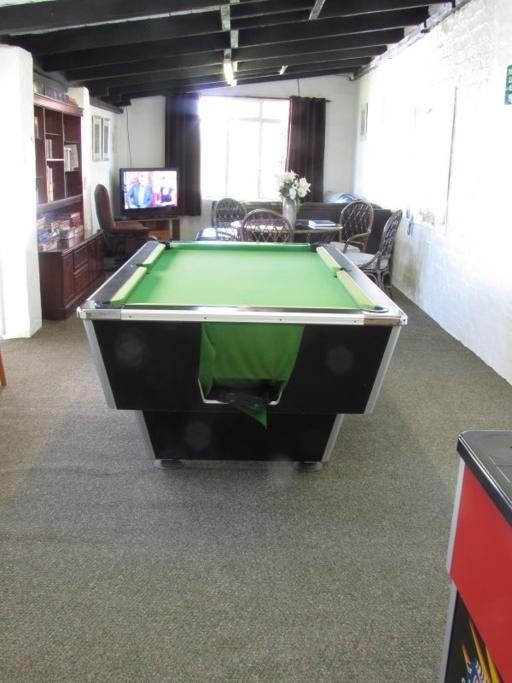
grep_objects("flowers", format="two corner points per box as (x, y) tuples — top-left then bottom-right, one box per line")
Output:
(278, 167), (311, 202)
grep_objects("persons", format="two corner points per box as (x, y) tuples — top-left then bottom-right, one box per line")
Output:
(125, 172), (177, 209)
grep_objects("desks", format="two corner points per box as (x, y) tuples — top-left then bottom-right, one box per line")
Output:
(76, 240), (408, 470)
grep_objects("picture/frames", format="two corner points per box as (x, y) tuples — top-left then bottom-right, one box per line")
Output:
(357, 102), (369, 137)
(91, 113), (110, 162)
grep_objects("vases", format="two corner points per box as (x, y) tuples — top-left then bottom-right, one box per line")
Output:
(280, 196), (298, 229)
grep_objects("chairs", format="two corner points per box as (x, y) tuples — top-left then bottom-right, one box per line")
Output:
(214, 199), (403, 299)
(94, 185), (150, 261)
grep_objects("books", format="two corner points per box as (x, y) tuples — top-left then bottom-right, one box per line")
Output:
(46, 138), (52, 159)
(34, 116), (40, 138)
(47, 164), (55, 202)
(308, 218), (343, 230)
(64, 143), (79, 172)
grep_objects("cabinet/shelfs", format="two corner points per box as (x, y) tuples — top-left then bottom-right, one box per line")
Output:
(33, 92), (105, 321)
(123, 216), (179, 243)
(437, 428), (512, 682)
